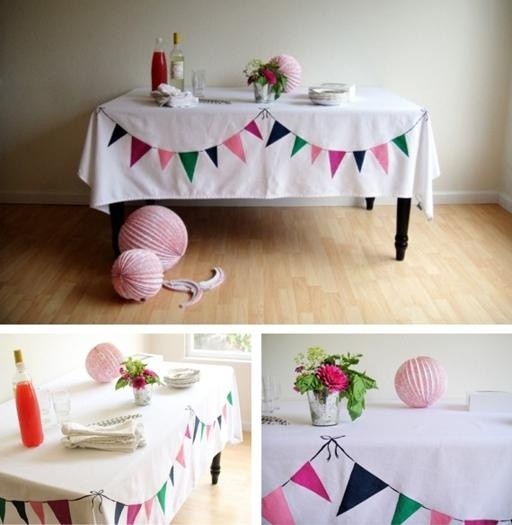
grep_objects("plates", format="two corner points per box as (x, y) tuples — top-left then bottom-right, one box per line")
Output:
(164, 369), (199, 388)
(306, 85), (347, 106)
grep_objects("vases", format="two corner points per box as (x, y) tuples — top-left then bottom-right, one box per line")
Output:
(132, 383), (153, 407)
(300, 386), (344, 428)
(252, 79), (274, 103)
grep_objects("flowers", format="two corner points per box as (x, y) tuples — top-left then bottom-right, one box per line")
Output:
(115, 356), (165, 391)
(242, 58), (288, 100)
(293, 346), (378, 422)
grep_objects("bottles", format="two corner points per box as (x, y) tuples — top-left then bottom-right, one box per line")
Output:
(150, 38), (167, 91)
(168, 31), (185, 92)
(14, 348), (44, 449)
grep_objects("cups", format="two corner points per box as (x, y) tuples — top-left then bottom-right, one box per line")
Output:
(191, 69), (206, 97)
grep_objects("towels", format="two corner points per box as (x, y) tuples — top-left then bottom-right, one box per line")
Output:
(63, 416), (147, 449)
(153, 82), (199, 109)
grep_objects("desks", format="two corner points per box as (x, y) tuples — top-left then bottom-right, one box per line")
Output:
(1, 359), (235, 524)
(98, 84), (426, 262)
(262, 388), (511, 525)
